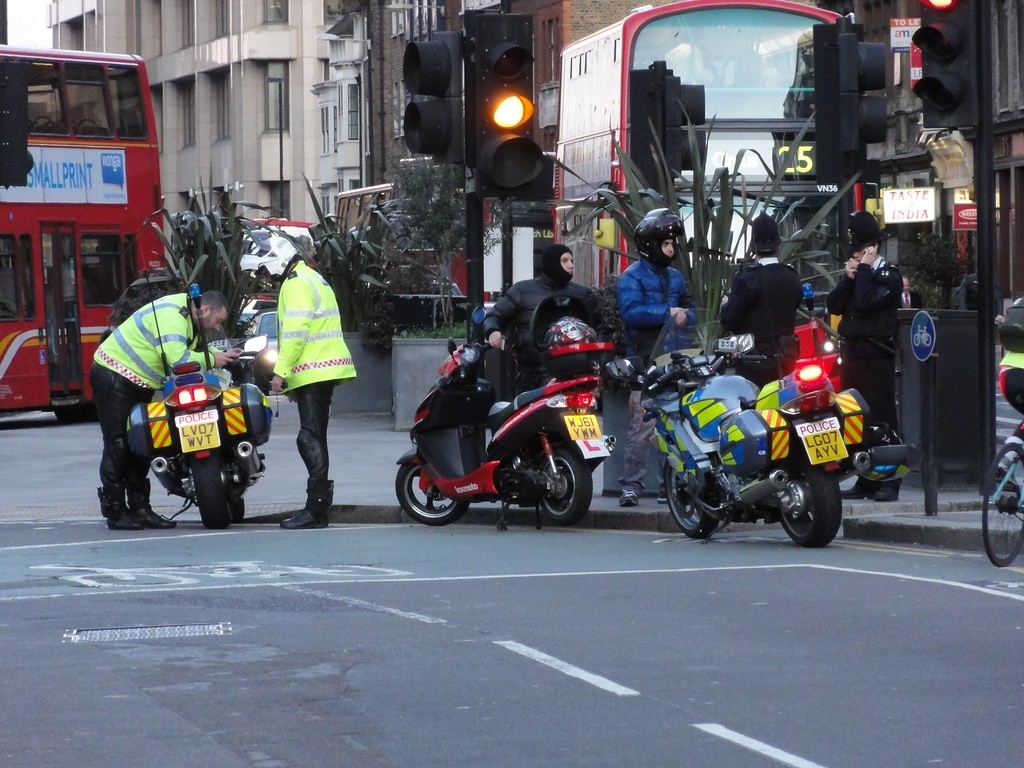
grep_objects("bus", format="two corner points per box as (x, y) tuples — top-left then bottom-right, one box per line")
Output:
(238, 220), (315, 316)
(560, 1), (861, 393)
(334, 183), (468, 302)
(0, 44), (163, 422)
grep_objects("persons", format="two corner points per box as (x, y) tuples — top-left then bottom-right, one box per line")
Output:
(89, 290), (242, 530)
(240, 228), (358, 530)
(483, 244), (614, 508)
(952, 262), (1004, 327)
(826, 208), (904, 502)
(718, 212), (804, 390)
(616, 208), (698, 507)
(898, 277), (923, 308)
(997, 349), (1024, 492)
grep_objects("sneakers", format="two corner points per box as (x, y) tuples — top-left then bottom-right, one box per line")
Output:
(619, 489), (638, 507)
(658, 492), (669, 504)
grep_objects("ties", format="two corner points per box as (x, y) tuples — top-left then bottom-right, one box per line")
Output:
(904, 293), (909, 308)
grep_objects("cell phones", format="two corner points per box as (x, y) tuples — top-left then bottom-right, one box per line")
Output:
(501, 336), (508, 351)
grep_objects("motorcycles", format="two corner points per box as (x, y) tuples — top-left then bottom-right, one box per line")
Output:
(607, 282), (872, 546)
(124, 284), (272, 529)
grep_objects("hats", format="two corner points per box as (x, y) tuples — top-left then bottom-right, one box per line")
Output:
(750, 210), (782, 251)
(847, 211), (890, 253)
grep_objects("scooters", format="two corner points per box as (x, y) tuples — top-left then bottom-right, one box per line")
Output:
(395, 302), (616, 530)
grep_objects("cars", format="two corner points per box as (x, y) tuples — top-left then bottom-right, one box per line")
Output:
(254, 311), (278, 378)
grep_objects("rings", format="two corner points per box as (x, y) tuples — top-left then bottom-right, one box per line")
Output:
(870, 253), (872, 254)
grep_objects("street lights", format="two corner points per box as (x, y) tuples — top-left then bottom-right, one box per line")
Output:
(266, 78), (283, 219)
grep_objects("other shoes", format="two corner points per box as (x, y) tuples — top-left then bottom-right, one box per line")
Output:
(840, 476), (902, 501)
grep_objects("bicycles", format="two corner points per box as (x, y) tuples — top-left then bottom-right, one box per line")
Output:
(982, 395), (1024, 566)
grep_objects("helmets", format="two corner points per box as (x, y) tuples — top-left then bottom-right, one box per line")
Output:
(240, 236), (298, 277)
(633, 214), (684, 264)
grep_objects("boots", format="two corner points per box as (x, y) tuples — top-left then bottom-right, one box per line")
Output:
(97, 481), (144, 530)
(280, 478), (334, 530)
(126, 478), (177, 529)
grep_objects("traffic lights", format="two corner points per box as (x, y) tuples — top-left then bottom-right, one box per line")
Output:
(473, 12), (546, 196)
(912, 0), (981, 129)
(838, 30), (888, 155)
(402, 30), (463, 164)
